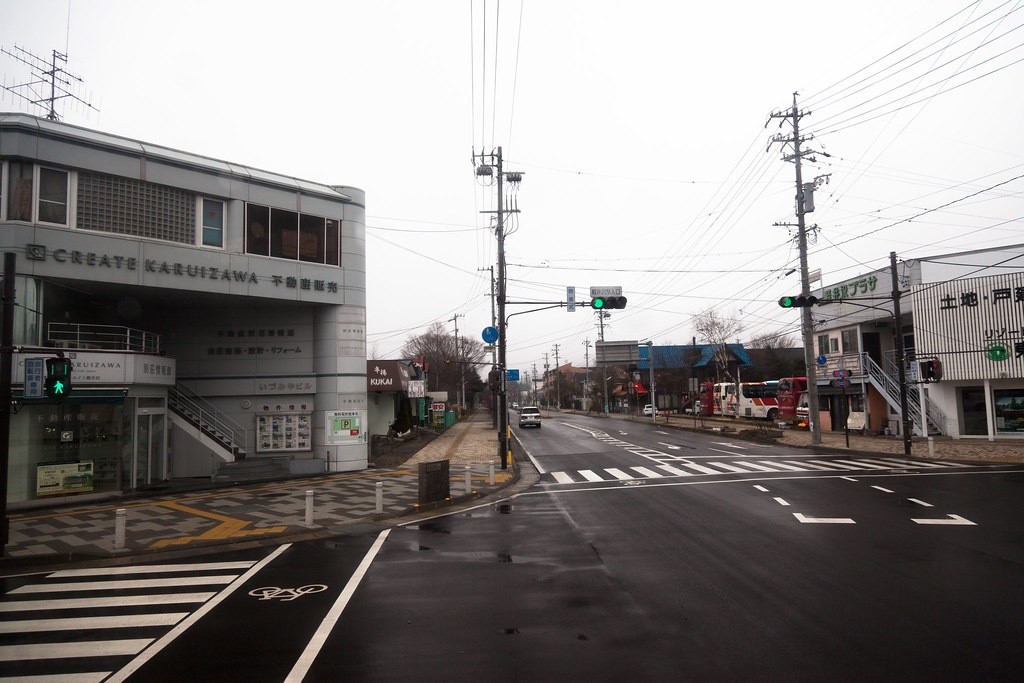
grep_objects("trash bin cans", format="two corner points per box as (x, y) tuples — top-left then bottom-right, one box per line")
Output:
(417, 459), (450, 504)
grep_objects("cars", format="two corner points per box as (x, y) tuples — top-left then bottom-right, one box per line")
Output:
(643, 404), (658, 417)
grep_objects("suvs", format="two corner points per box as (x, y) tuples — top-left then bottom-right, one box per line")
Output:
(517, 406), (542, 428)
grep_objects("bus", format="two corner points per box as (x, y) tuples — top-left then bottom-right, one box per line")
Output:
(714, 382), (738, 419)
(997, 408), (1024, 428)
(777, 378), (808, 424)
(698, 381), (724, 415)
(737, 381), (778, 421)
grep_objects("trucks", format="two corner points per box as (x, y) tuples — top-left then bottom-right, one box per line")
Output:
(686, 401), (700, 415)
(797, 390), (810, 429)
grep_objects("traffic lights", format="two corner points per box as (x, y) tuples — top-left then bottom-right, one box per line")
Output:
(590, 296), (627, 309)
(48, 357), (73, 399)
(932, 361), (943, 379)
(779, 296), (818, 308)
